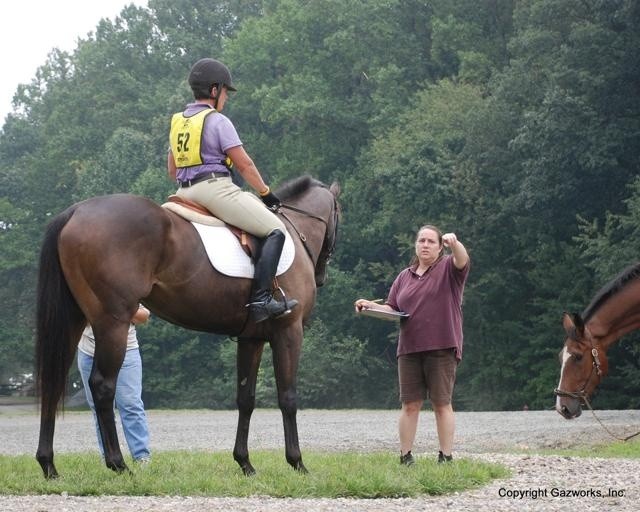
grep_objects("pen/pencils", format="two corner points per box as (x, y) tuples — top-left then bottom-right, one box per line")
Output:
(353, 298), (384, 306)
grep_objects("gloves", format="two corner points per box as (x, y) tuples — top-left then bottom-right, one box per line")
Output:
(259, 187), (279, 207)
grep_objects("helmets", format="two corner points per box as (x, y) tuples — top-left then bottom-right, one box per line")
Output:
(189, 59), (237, 91)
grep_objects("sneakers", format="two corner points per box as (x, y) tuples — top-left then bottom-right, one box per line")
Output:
(401, 451), (453, 465)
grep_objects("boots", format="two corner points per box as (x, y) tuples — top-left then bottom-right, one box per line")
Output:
(249, 229), (298, 325)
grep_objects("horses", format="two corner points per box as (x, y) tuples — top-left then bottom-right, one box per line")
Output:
(29, 171), (346, 481)
(552, 256), (640, 422)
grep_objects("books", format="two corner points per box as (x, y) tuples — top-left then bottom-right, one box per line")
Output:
(360, 309), (409, 320)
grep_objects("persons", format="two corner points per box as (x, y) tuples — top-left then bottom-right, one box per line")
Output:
(355, 225), (471, 465)
(77, 303), (151, 463)
(167, 57), (298, 325)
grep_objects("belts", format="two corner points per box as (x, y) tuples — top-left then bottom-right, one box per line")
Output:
(179, 171), (229, 187)
